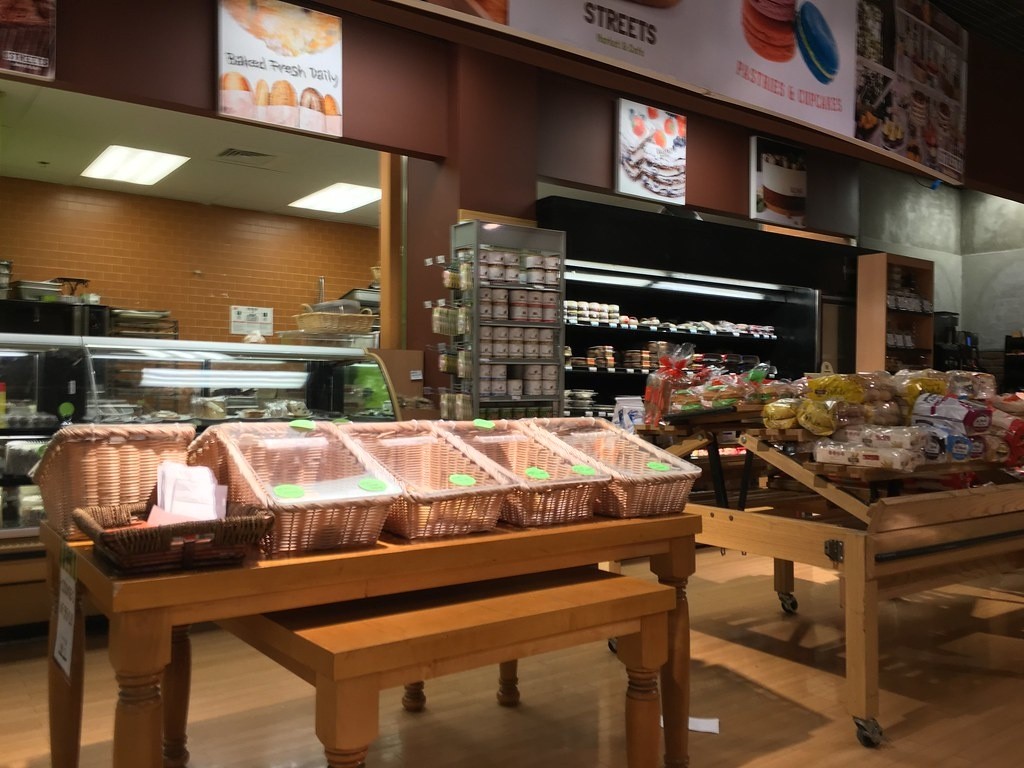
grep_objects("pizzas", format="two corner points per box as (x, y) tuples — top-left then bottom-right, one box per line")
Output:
(224, 0), (342, 57)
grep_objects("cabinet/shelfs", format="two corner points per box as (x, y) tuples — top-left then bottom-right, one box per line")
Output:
(0, 300), (110, 337)
(856, 252), (935, 373)
(110, 315), (179, 339)
(634, 411), (824, 510)
(1, 333), (403, 529)
(421, 218), (566, 420)
(737, 427), (1024, 533)
(565, 259), (821, 422)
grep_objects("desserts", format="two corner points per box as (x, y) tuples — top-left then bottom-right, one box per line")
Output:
(740, 0), (839, 84)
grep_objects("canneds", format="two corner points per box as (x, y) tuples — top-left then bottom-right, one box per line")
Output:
(457, 248), (560, 419)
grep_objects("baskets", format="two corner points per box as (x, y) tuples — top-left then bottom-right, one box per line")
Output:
(31, 417), (703, 579)
(291, 303), (379, 333)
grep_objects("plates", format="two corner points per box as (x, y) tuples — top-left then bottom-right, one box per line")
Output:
(111, 309), (171, 318)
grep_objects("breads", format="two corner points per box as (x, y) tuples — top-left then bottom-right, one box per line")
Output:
(222, 72), (339, 116)
(760, 375), (920, 436)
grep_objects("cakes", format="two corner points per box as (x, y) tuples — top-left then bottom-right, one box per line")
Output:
(620, 100), (685, 199)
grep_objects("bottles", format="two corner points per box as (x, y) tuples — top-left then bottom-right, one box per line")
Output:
(0, 382), (8, 427)
(63, 379), (81, 423)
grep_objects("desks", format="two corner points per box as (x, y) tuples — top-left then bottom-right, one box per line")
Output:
(162, 566), (677, 768)
(37, 511), (704, 768)
